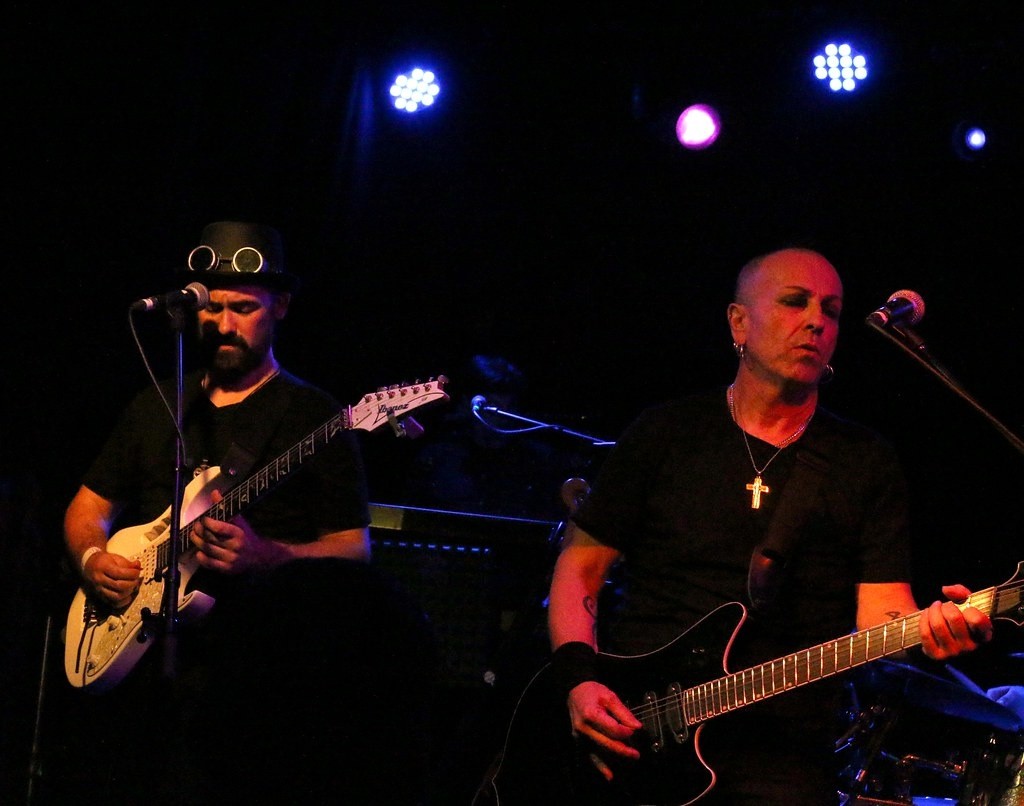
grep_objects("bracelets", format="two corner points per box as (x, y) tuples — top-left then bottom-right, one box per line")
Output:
(551, 639), (600, 696)
(80, 546), (101, 577)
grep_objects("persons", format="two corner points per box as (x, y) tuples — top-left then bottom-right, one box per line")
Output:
(61, 215), (404, 806)
(548, 243), (995, 806)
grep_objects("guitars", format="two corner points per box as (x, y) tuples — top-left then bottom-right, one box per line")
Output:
(489, 559), (1024, 806)
(61, 371), (454, 698)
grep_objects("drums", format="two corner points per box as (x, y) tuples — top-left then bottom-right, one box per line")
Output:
(895, 754), (963, 806)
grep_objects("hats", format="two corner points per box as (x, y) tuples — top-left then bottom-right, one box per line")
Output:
(174, 220), (301, 294)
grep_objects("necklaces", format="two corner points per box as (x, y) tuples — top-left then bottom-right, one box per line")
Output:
(191, 366), (280, 479)
(727, 380), (816, 510)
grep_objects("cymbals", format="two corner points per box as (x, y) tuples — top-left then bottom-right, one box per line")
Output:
(843, 659), (1023, 730)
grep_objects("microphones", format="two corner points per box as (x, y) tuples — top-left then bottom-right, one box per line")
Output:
(869, 289), (926, 329)
(137, 281), (211, 312)
(470, 395), (488, 411)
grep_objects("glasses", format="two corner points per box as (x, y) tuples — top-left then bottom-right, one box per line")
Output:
(188, 246), (273, 274)
